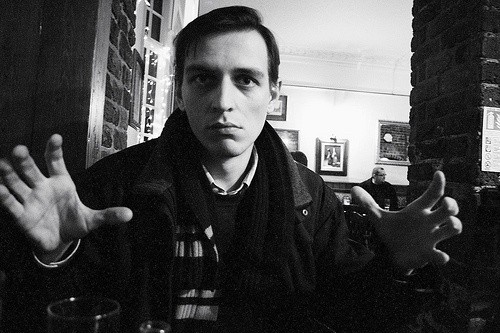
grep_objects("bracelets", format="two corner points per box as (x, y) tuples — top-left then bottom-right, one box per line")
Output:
(29, 237), (82, 269)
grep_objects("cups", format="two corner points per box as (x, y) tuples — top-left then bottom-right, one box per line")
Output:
(343, 196), (351, 205)
(48, 296), (121, 333)
(384, 199), (390, 208)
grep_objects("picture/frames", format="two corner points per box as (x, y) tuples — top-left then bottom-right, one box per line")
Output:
(266, 94), (287, 121)
(376, 120), (409, 165)
(275, 128), (299, 151)
(316, 138), (350, 177)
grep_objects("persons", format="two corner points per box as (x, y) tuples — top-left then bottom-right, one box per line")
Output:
(359, 169), (398, 211)
(0, 6), (462, 333)
